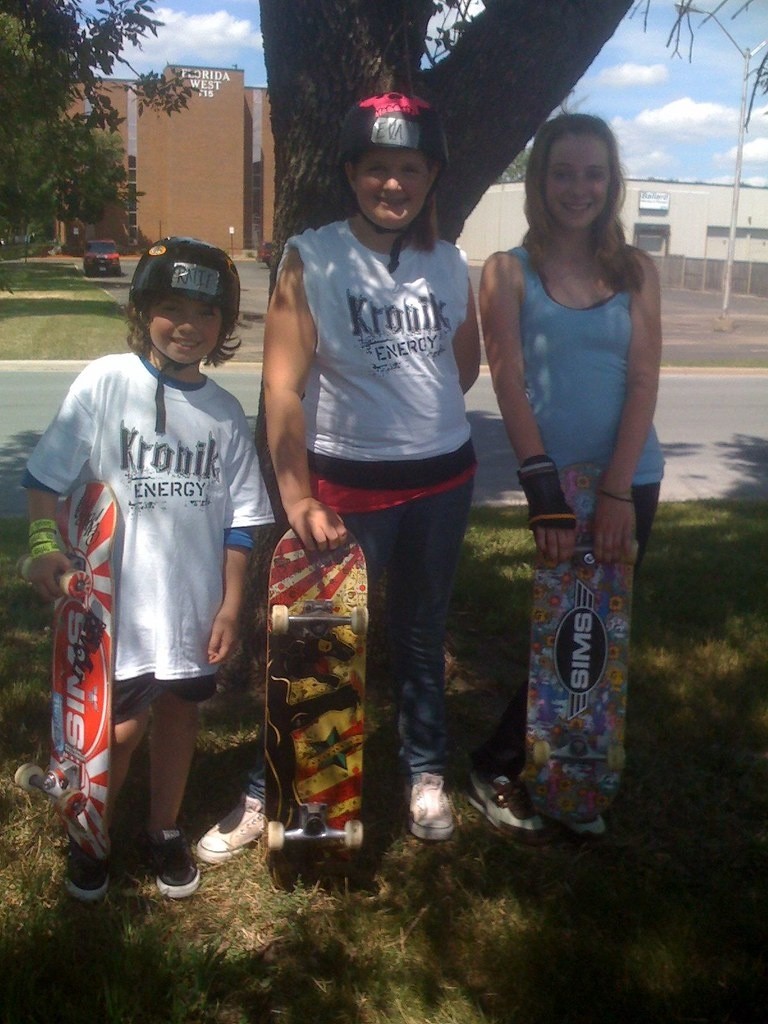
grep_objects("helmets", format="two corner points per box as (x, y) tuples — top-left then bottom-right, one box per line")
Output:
(131, 236), (239, 320)
(343, 91), (449, 178)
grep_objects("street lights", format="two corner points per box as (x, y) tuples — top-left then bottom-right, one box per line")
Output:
(676, 2), (761, 308)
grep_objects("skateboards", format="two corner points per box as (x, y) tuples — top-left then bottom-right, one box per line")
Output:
(14, 479), (120, 862)
(523, 463), (641, 823)
(263, 525), (372, 896)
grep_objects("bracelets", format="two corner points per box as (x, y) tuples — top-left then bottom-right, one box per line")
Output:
(26, 517), (64, 560)
(597, 486), (636, 502)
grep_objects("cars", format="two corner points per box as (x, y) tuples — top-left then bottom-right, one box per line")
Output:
(257, 242), (272, 268)
(84, 239), (122, 276)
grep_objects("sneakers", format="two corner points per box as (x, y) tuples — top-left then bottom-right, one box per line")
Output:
(64, 834), (109, 901)
(196, 791), (270, 865)
(142, 823), (200, 901)
(466, 770), (550, 846)
(403, 773), (454, 842)
(568, 815), (608, 839)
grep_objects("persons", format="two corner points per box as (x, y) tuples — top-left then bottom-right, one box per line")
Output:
(466, 112), (667, 844)
(20, 236), (276, 903)
(259, 92), (481, 844)
